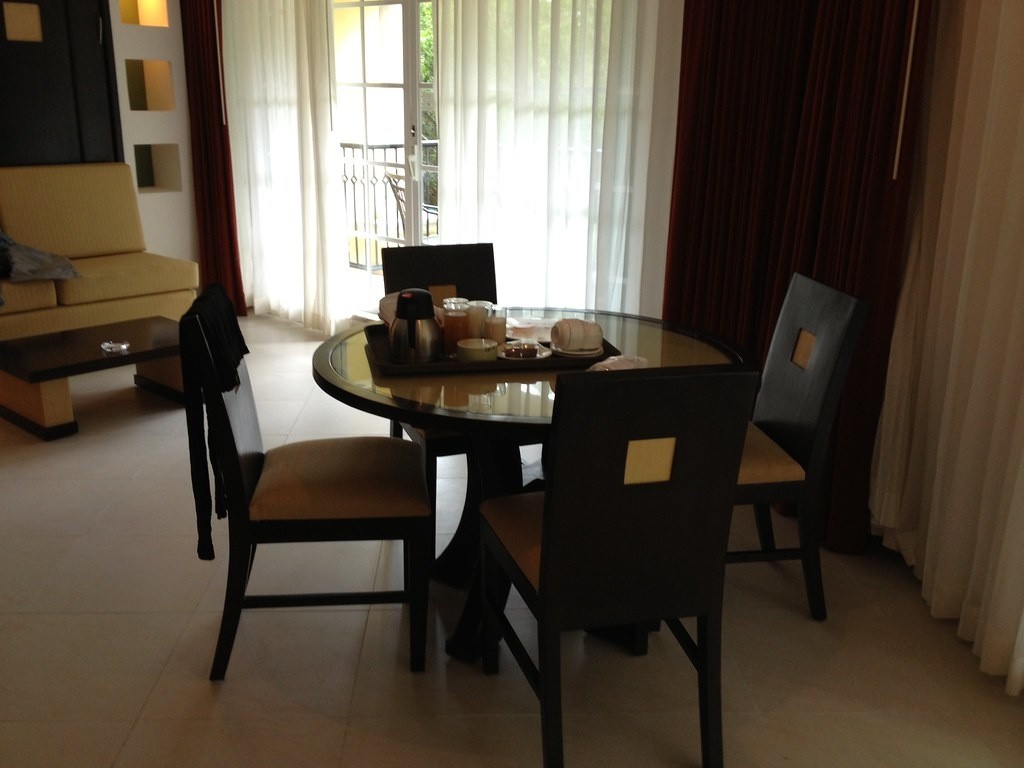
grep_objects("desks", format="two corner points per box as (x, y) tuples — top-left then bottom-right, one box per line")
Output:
(312, 307), (763, 678)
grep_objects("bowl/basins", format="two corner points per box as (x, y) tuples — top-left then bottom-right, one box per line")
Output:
(456, 338), (498, 362)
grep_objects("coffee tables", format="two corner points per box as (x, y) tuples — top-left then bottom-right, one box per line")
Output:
(0, 316), (207, 442)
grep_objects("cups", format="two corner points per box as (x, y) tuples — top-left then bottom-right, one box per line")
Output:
(442, 298), (506, 358)
(551, 318), (603, 352)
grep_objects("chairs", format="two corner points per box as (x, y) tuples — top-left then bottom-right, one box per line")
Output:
(468, 361), (767, 768)
(383, 172), (439, 246)
(726, 272), (860, 623)
(177, 279), (431, 685)
(382, 242), (549, 484)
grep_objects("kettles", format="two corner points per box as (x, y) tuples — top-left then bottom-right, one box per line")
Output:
(388, 288), (442, 364)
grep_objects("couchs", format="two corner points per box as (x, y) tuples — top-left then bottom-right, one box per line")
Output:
(0, 162), (200, 343)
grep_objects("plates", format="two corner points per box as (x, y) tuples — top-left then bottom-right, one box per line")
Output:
(505, 316), (559, 343)
(550, 343), (604, 358)
(496, 344), (552, 359)
